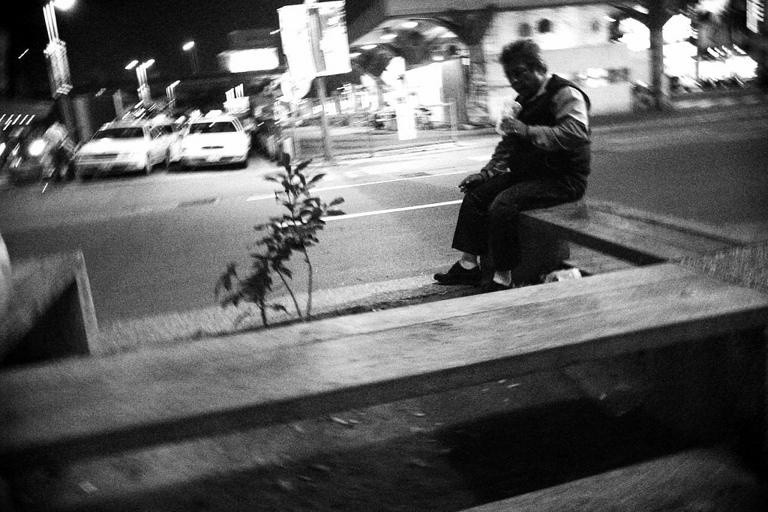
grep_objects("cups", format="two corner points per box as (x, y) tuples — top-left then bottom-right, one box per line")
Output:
(494, 98), (523, 137)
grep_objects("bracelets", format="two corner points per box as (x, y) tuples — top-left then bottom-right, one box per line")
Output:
(525, 126), (529, 135)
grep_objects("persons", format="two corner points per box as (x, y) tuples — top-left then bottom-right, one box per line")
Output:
(432, 40), (594, 291)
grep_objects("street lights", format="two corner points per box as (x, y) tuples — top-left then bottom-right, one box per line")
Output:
(136, 58), (155, 107)
(42, 1), (80, 146)
(166, 79), (182, 116)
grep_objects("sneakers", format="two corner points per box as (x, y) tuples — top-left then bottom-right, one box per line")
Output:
(434, 260), (509, 291)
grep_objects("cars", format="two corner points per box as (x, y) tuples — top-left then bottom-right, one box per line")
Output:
(180, 115), (252, 170)
(0, 128), (70, 182)
(76, 121), (187, 181)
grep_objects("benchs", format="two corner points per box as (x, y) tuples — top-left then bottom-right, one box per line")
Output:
(2, 246), (99, 373)
(525, 195), (768, 276)
(2, 240), (767, 444)
